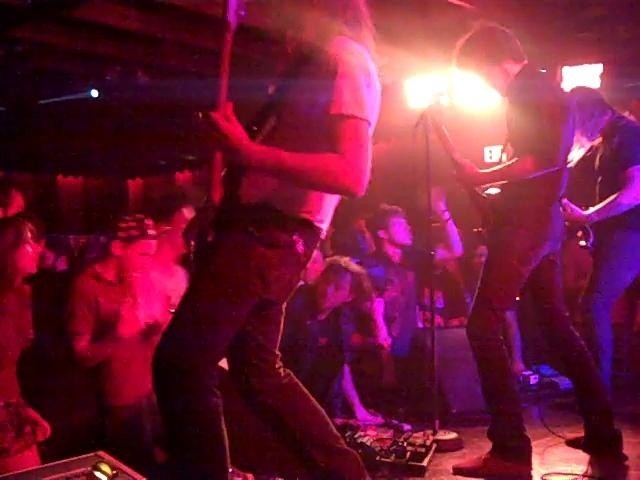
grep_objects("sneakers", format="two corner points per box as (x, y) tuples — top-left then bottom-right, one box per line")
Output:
(564, 430), (623, 458)
(453, 446), (533, 476)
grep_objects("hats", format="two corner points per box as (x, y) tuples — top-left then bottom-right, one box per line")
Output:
(107, 213), (176, 241)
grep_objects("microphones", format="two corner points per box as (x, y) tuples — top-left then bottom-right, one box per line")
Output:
(415, 103), (437, 128)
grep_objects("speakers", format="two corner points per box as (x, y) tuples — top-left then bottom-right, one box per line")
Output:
(411, 327), (492, 425)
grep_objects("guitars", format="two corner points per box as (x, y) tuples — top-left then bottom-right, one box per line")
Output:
(433, 115), (498, 244)
(558, 197), (594, 293)
(184, 0), (246, 269)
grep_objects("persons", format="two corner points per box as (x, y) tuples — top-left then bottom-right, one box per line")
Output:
(0, 1), (640, 480)
